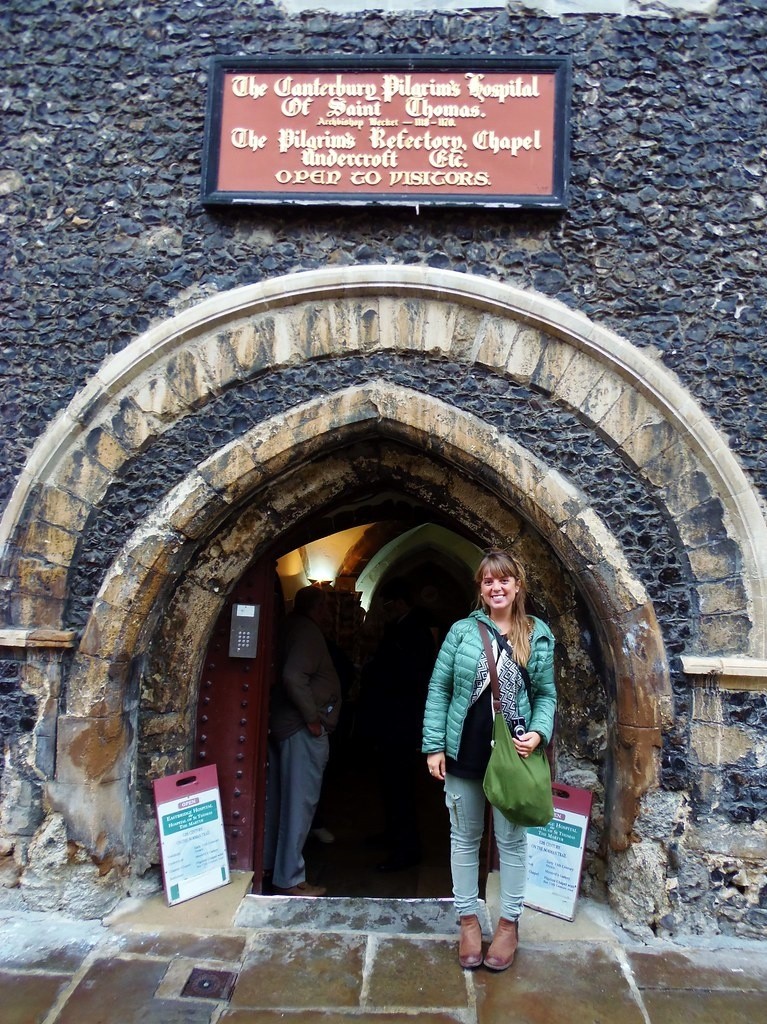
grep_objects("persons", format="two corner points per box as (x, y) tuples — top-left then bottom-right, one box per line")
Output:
(263, 586), (343, 896)
(349, 576), (436, 874)
(421, 547), (557, 971)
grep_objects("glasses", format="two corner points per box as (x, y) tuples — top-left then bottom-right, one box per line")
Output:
(383, 600), (393, 610)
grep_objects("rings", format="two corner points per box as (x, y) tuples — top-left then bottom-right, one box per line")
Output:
(429, 769), (434, 774)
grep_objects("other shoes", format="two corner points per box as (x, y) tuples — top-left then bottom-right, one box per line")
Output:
(272, 882), (326, 896)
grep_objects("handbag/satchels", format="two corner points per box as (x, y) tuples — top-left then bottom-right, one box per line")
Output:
(483, 712), (556, 827)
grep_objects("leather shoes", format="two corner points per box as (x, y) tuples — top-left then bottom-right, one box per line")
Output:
(459, 914), (483, 968)
(483, 917), (518, 970)
